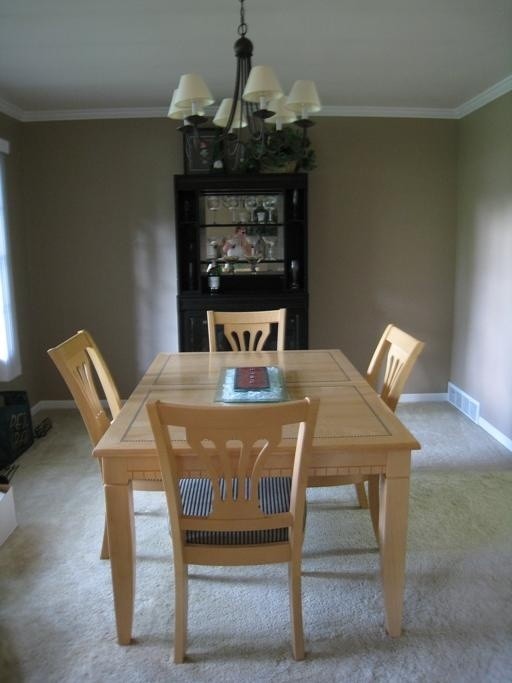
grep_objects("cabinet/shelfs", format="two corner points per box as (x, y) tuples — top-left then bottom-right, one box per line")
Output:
(173, 172), (310, 354)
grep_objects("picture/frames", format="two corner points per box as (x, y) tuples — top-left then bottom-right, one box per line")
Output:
(182, 128), (227, 176)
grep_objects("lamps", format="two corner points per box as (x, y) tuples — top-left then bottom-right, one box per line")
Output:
(168, 0), (324, 157)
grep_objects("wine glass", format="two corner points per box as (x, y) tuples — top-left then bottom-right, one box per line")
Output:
(207, 194), (221, 225)
(244, 235), (262, 261)
(262, 195), (278, 225)
(262, 236), (278, 261)
(244, 195), (260, 225)
(224, 236), (240, 261)
(225, 195), (241, 225)
(208, 236), (223, 260)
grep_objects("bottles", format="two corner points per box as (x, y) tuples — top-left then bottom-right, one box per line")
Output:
(207, 260), (221, 296)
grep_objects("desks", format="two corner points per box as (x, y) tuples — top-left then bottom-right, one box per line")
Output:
(90, 351), (423, 644)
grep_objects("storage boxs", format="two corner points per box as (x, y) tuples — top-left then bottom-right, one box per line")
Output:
(1, 392), (36, 466)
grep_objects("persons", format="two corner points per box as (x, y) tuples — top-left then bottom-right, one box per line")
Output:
(222, 219), (253, 266)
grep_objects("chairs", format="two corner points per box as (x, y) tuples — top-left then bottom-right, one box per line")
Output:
(48, 329), (165, 556)
(205, 308), (284, 352)
(310, 324), (425, 544)
(142, 394), (321, 667)
